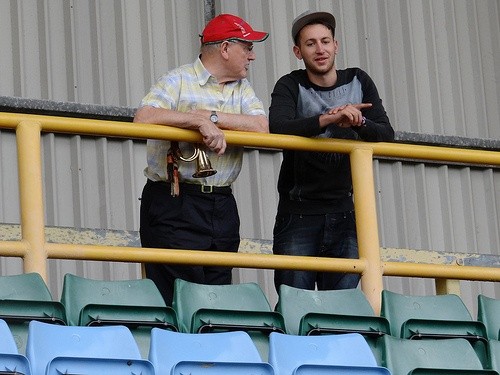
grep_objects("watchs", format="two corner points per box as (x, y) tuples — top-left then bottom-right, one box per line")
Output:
(210, 111), (218, 125)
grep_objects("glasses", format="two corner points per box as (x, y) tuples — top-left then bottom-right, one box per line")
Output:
(243, 46), (253, 51)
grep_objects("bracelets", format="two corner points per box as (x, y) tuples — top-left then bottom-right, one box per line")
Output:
(362, 117), (367, 126)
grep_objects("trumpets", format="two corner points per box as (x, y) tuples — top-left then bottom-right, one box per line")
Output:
(179, 103), (218, 178)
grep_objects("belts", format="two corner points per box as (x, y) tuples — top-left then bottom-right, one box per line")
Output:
(165, 181), (231, 195)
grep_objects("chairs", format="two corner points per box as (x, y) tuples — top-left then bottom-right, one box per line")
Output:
(382, 334), (500, 375)
(59, 273), (181, 361)
(476, 292), (500, 370)
(0, 318), (33, 375)
(273, 283), (391, 369)
(170, 278), (287, 365)
(487, 338), (500, 375)
(268, 333), (392, 375)
(0, 272), (68, 357)
(379, 288), (492, 371)
(25, 320), (156, 375)
(147, 327), (276, 375)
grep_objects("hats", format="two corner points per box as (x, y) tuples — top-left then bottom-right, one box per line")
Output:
(199, 14), (269, 44)
(292, 10), (336, 45)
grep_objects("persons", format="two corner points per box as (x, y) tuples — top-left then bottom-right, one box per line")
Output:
(132, 14), (271, 306)
(268, 10), (394, 311)
